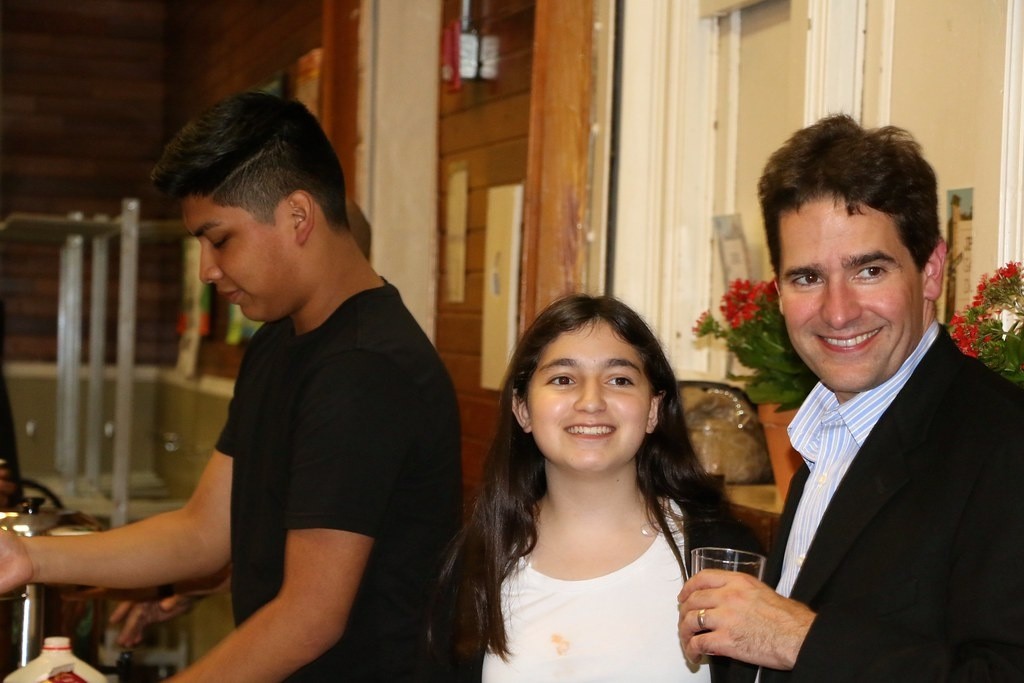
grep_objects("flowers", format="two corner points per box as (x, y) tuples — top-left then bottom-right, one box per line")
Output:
(948, 261), (1024, 360)
(688, 274), (777, 338)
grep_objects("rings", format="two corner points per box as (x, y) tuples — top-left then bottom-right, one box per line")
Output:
(697, 608), (707, 630)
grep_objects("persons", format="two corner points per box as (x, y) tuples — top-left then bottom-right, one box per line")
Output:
(421, 292), (768, 683)
(675, 112), (1024, 683)
(0, 86), (467, 682)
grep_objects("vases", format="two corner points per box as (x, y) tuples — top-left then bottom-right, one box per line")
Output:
(758, 403), (802, 504)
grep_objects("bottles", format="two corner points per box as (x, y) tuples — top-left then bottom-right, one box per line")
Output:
(0, 634), (108, 683)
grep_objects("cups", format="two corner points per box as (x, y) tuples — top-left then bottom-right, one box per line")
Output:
(690, 546), (766, 655)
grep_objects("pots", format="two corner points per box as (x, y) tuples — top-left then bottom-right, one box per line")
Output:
(1, 495), (110, 679)
